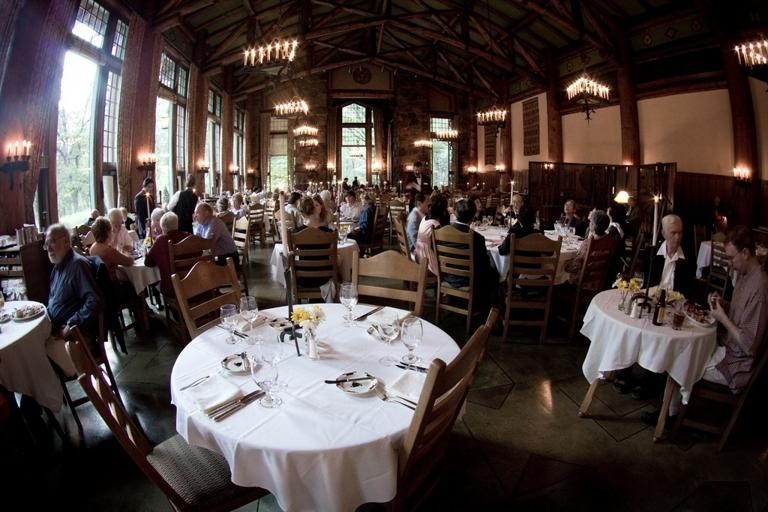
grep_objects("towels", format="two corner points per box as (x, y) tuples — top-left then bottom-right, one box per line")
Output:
(236, 314), (248, 331)
(385, 369), (427, 404)
(189, 375), (241, 414)
(320, 280), (336, 304)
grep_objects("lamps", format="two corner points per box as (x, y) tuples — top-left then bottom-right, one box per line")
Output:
(299, 137), (318, 148)
(437, 127), (458, 142)
(413, 134), (433, 150)
(135, 152), (157, 172)
(243, 12), (297, 97)
(564, 34), (609, 125)
(734, 30), (768, 84)
(292, 118), (318, 140)
(1, 139), (33, 173)
(271, 69), (308, 125)
(475, 14), (507, 134)
(732, 164), (753, 188)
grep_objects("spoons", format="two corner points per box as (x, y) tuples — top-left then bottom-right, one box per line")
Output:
(376, 386), (418, 406)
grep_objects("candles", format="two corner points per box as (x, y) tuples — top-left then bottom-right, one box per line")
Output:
(652, 196), (658, 245)
(510, 181), (513, 204)
(147, 194), (150, 218)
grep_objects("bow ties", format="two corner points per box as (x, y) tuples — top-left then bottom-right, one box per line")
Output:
(415, 176), (420, 178)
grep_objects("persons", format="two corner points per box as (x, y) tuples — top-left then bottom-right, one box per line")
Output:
(140, 207), (166, 240)
(441, 199), (500, 288)
(352, 177), (359, 187)
(144, 213), (202, 304)
(89, 217), (151, 343)
(320, 182), (332, 203)
(134, 178), (155, 238)
(706, 196), (725, 234)
(606, 205), (624, 265)
(430, 185), (439, 197)
(498, 206), (555, 292)
(467, 196), (486, 223)
(352, 176), (360, 186)
(487, 186), (497, 198)
(288, 197), (335, 289)
(86, 209), (99, 227)
(312, 194), (336, 229)
(278, 192), (304, 233)
(347, 190), (377, 253)
(214, 182), (284, 218)
(119, 207), (133, 230)
(625, 197), (641, 234)
(359, 183), (380, 193)
(194, 202), (240, 285)
(564, 210), (616, 299)
(107, 208), (134, 253)
(412, 194), (450, 276)
(639, 223), (767, 433)
(500, 194), (535, 229)
(552, 199), (588, 236)
(287, 183), (312, 196)
(584, 206), (604, 238)
(611, 213), (697, 401)
(166, 173), (198, 232)
(342, 177), (351, 192)
(405, 192), (431, 253)
(440, 185), (447, 194)
(405, 161), (432, 213)
(382, 181), (391, 193)
(19, 223), (98, 377)
(340, 191), (361, 222)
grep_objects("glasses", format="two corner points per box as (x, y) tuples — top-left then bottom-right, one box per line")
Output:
(42, 234), (62, 251)
(727, 250), (739, 261)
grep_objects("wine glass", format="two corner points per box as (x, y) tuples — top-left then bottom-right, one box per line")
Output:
(339, 283), (358, 319)
(0, 291), (6, 317)
(240, 296), (263, 344)
(220, 304), (239, 344)
(399, 318), (423, 364)
(341, 286), (357, 326)
(251, 361), (282, 409)
(635, 271), (643, 291)
(379, 311), (399, 367)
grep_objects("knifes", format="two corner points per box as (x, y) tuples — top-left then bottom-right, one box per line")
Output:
(325, 377), (376, 383)
(213, 392), (265, 422)
(208, 390), (259, 418)
(354, 306), (385, 321)
(216, 326), (245, 339)
(396, 365), (427, 373)
(400, 362), (428, 370)
(236, 331), (248, 337)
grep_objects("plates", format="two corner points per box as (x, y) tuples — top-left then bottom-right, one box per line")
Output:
(10, 305), (44, 321)
(222, 354), (257, 372)
(336, 372), (377, 395)
(268, 317), (289, 329)
(683, 303), (715, 327)
(367, 323), (399, 341)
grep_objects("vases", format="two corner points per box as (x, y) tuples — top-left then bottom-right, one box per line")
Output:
(617, 296), (628, 310)
(307, 335), (319, 360)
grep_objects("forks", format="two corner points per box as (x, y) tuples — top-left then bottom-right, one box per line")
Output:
(375, 388), (416, 411)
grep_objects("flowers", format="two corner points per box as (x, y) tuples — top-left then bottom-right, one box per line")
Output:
(612, 276), (641, 295)
(290, 304), (326, 337)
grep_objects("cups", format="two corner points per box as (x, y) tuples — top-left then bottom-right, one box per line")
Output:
(673, 302), (689, 330)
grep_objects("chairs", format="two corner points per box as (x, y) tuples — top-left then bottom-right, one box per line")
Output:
(171, 256), (246, 339)
(65, 326), (271, 512)
(286, 227), (341, 303)
(83, 255), (148, 342)
(352, 250), (429, 320)
(163, 234), (220, 343)
(217, 212), (240, 237)
(430, 224), (484, 337)
(265, 200), (276, 242)
(672, 349), (768, 449)
(385, 306), (498, 512)
(693, 224), (706, 258)
(273, 210), (295, 242)
(232, 216), (250, 280)
(392, 215), (449, 316)
(358, 206), (389, 255)
(705, 232), (730, 298)
(498, 234), (562, 344)
(248, 203), (264, 249)
(565, 234), (620, 333)
(622, 232), (648, 284)
(58, 254), (123, 424)
(493, 204), (510, 227)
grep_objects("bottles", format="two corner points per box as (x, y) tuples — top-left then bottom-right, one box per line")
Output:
(630, 298), (638, 319)
(307, 330), (320, 360)
(624, 295), (632, 314)
(652, 288), (666, 326)
